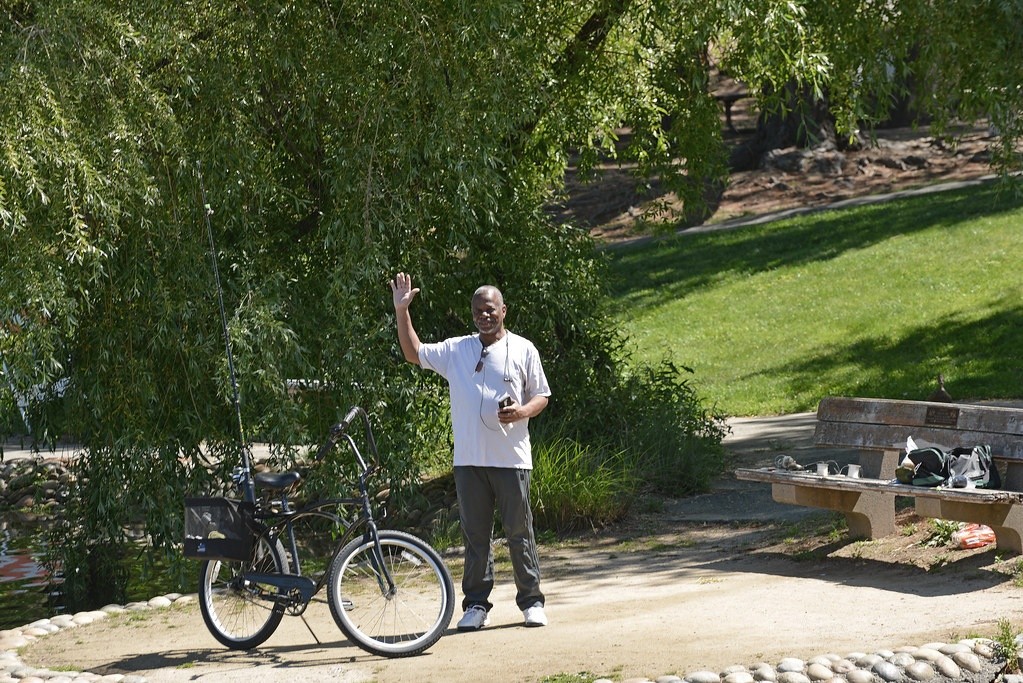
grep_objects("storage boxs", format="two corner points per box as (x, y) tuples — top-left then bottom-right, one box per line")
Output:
(184, 496), (254, 561)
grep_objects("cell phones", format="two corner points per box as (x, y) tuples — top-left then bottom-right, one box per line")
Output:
(497, 395), (514, 414)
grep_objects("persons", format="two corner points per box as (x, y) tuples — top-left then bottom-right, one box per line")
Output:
(390, 272), (552, 629)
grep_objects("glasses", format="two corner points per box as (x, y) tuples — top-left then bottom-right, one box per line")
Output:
(475, 346), (489, 372)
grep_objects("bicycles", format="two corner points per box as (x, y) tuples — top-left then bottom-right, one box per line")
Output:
(198, 407), (455, 658)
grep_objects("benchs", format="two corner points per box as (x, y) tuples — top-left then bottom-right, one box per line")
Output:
(738, 396), (1023, 556)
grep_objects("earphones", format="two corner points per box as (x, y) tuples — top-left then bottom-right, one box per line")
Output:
(504, 378), (508, 382)
(509, 377), (513, 382)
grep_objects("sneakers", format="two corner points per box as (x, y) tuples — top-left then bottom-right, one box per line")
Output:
(457, 604), (491, 631)
(522, 601), (548, 627)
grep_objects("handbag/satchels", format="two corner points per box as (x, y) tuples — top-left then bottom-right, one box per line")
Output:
(895, 435), (918, 485)
(908, 443), (1002, 490)
